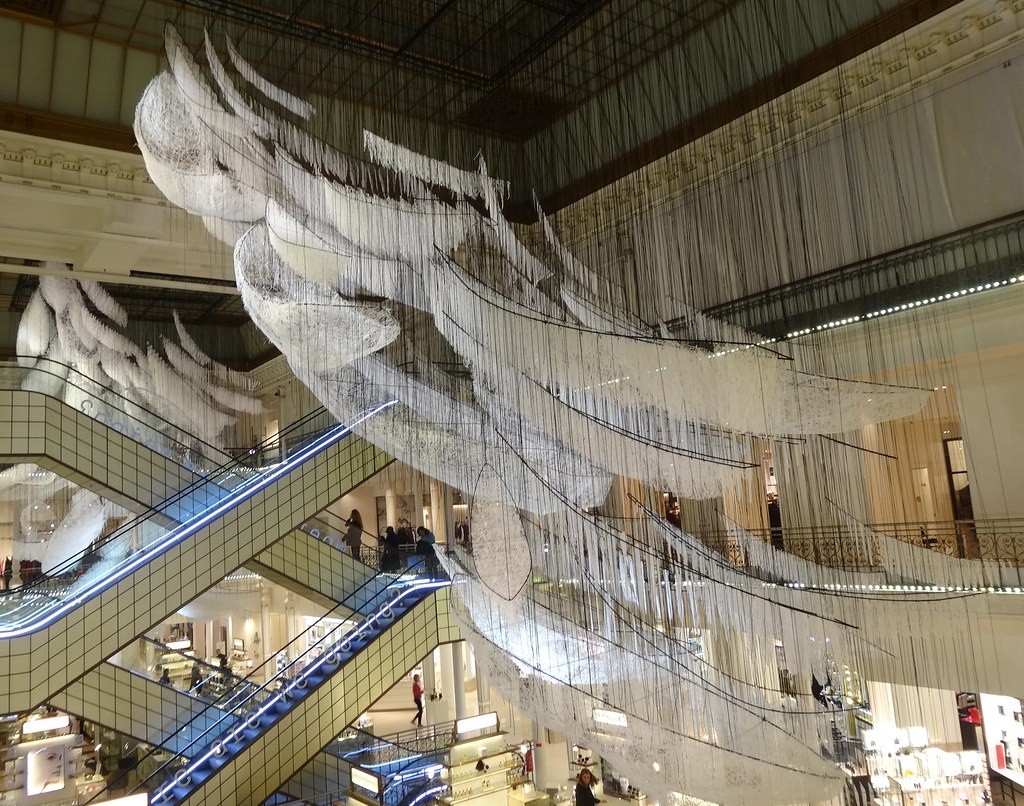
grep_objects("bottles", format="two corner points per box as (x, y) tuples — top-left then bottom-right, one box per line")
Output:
(452, 769), (473, 800)
(463, 754), (467, 761)
(494, 743), (520, 785)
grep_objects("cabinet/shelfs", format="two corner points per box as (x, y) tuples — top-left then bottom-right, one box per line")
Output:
(566, 743), (648, 806)
(155, 637), (254, 708)
(435, 711), (553, 806)
(859, 724), (994, 806)
(136, 743), (188, 789)
(0, 712), (110, 806)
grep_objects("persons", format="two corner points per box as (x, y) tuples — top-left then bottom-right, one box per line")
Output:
(159, 669), (173, 686)
(162, 638), (166, 645)
(455, 521), (459, 543)
(575, 769), (607, 806)
(190, 666), (203, 694)
(216, 649), (232, 684)
(33, 749), (62, 791)
(416, 526), (439, 583)
(342, 509), (363, 561)
(45, 706), (58, 717)
(379, 527), (402, 573)
(411, 674), (424, 727)
(399, 525), (414, 552)
(2, 556), (14, 590)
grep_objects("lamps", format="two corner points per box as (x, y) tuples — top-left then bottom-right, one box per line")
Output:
(92, 744), (103, 782)
(520, 738), (534, 781)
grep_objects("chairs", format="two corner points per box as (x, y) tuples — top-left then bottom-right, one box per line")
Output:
(105, 769), (129, 797)
